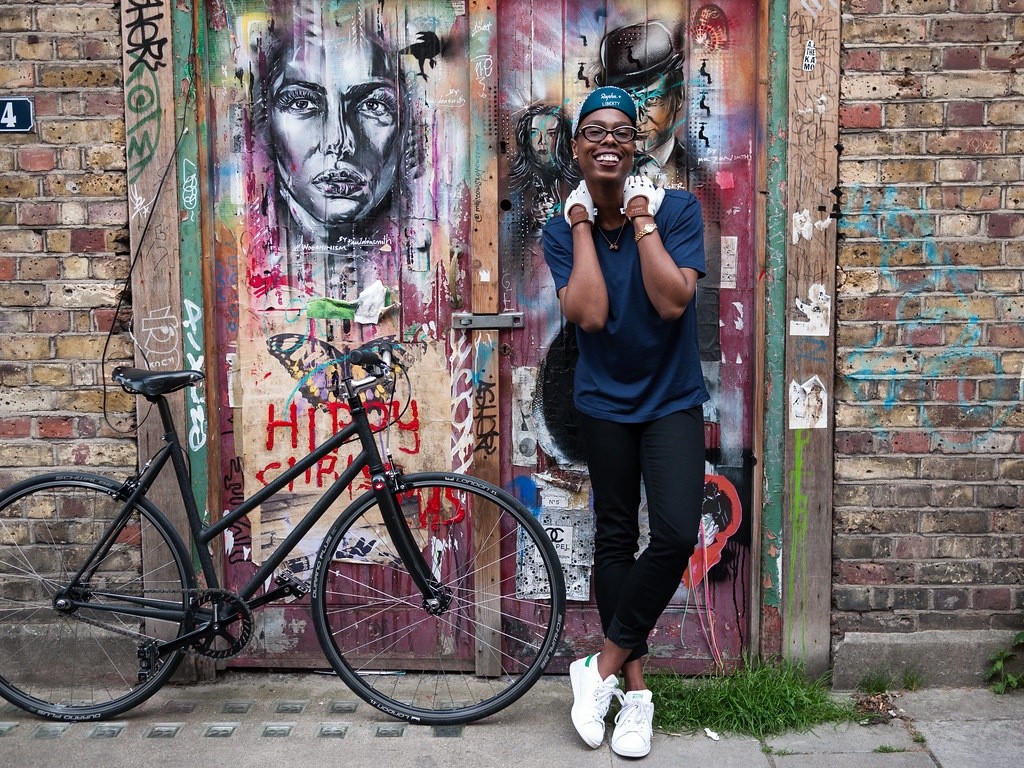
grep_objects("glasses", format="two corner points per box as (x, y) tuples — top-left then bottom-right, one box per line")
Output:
(574, 125), (639, 143)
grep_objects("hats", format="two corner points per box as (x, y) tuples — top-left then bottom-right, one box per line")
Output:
(579, 85), (637, 124)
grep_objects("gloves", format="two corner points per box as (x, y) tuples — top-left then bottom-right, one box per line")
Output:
(563, 180), (598, 230)
(619, 175), (666, 221)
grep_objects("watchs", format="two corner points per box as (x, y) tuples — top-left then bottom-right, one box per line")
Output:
(634, 224), (658, 243)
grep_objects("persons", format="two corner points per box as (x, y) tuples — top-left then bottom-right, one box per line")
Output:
(544, 85), (709, 758)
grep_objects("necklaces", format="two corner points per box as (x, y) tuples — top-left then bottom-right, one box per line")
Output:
(596, 214), (627, 250)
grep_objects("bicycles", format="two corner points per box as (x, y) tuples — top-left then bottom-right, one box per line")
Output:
(0, 335), (567, 726)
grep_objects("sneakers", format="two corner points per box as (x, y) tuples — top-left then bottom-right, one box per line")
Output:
(569, 652), (628, 749)
(610, 689), (655, 758)
